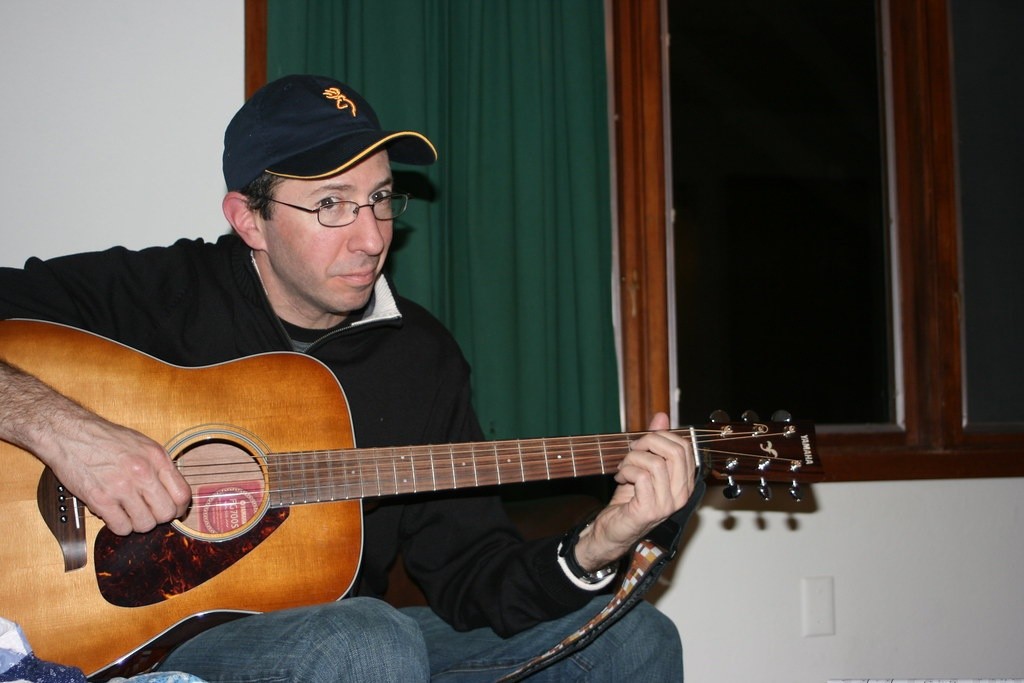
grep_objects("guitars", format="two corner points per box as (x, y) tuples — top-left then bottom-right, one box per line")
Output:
(0, 317), (825, 681)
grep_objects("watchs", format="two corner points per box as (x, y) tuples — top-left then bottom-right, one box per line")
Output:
(559, 517), (619, 584)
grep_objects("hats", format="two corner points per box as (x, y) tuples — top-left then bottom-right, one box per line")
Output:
(223, 75), (438, 197)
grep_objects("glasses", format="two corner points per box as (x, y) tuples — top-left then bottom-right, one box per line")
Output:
(250, 193), (411, 228)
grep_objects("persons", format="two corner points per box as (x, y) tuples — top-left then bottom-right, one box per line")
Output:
(0, 75), (694, 683)
